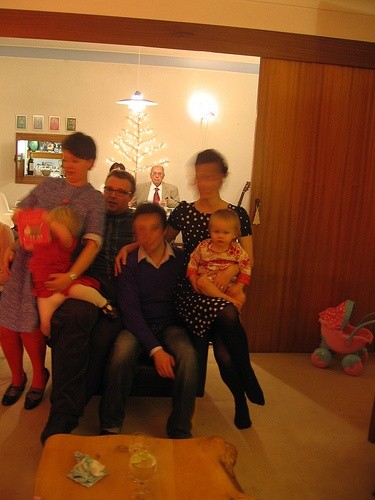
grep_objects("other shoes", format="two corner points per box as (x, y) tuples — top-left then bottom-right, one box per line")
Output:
(168, 430), (192, 438)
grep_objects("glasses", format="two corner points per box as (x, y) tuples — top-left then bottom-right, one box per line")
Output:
(104, 185), (131, 197)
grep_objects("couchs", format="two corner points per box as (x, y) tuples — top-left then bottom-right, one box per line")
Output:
(51, 247), (206, 398)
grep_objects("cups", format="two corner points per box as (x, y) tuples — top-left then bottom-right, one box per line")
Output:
(29, 141), (39, 152)
(167, 208), (175, 212)
(39, 141), (54, 152)
(35, 164), (50, 176)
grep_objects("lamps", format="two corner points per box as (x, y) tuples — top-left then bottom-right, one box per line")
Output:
(116, 46), (156, 115)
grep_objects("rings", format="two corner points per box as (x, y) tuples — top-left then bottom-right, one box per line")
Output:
(220, 286), (224, 289)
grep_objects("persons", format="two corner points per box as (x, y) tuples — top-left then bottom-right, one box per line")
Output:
(12, 209), (118, 348)
(114, 149), (265, 429)
(40, 171), (136, 444)
(128, 166), (180, 211)
(187, 209), (251, 312)
(109, 163), (125, 172)
(0, 132), (105, 410)
(99, 203), (198, 439)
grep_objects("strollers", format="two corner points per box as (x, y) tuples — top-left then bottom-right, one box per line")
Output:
(310, 301), (375, 376)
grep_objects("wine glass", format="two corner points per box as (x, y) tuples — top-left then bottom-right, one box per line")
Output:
(127, 431), (160, 500)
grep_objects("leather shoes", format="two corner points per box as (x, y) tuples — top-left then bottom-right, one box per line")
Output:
(45, 335), (51, 347)
(100, 299), (118, 320)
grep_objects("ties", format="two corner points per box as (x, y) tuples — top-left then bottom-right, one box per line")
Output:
(153, 188), (160, 205)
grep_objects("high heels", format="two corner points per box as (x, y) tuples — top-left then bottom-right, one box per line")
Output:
(2, 372), (27, 406)
(24, 368), (49, 409)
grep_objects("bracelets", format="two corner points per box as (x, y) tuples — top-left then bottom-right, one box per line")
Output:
(149, 345), (163, 357)
(68, 272), (77, 280)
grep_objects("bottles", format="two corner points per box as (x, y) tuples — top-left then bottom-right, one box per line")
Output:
(46, 163), (52, 171)
(28, 151), (34, 175)
(18, 155), (21, 177)
(54, 143), (59, 153)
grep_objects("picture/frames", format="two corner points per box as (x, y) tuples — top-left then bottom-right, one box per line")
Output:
(16, 115), (27, 129)
(32, 115), (44, 130)
(49, 116), (60, 131)
(65, 117), (77, 131)
